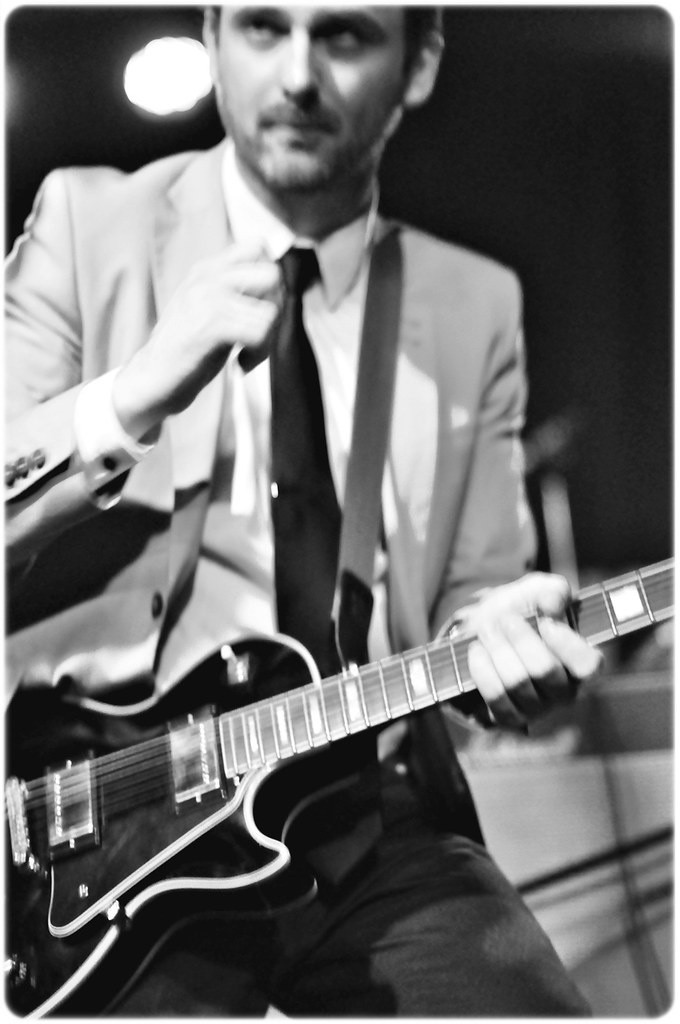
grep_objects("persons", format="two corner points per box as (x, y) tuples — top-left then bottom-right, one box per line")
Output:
(4, 5), (609, 1019)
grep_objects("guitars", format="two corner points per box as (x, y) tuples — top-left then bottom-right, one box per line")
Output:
(6, 558), (675, 1018)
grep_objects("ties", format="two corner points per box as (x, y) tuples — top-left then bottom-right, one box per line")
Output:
(266, 248), (341, 673)
